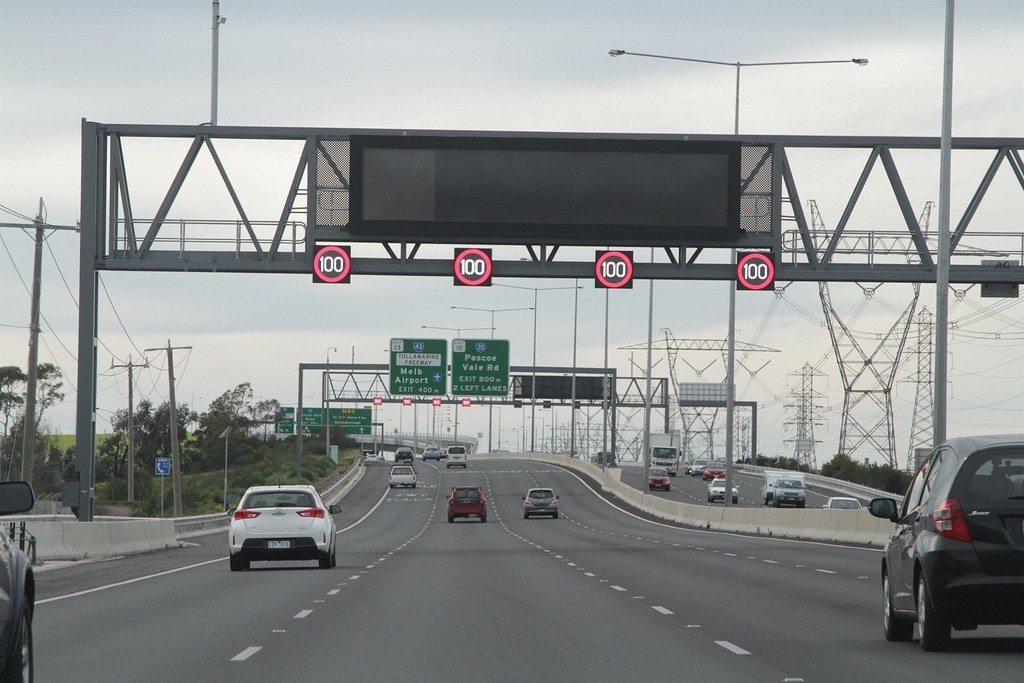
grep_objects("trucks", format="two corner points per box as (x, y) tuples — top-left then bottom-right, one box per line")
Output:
(762, 470), (805, 505)
(648, 433), (684, 477)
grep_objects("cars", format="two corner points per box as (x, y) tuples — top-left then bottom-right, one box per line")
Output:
(388, 465), (417, 489)
(365, 454), (380, 463)
(866, 433), (1024, 653)
(1, 480), (39, 683)
(521, 488), (561, 520)
(646, 466), (672, 493)
(822, 497), (863, 509)
(394, 447), (414, 462)
(422, 447), (439, 461)
(439, 447), (448, 459)
(228, 485), (343, 570)
(707, 477), (740, 504)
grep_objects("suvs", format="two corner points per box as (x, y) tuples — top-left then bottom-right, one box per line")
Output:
(446, 486), (488, 523)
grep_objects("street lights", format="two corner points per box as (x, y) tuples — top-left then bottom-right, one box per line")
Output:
(605, 44), (873, 498)
(487, 282), (585, 454)
(325, 346), (337, 459)
(451, 304), (534, 453)
(218, 424), (232, 513)
(420, 325), (498, 445)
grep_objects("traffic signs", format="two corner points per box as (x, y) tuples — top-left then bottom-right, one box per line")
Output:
(451, 337), (510, 399)
(387, 337), (449, 398)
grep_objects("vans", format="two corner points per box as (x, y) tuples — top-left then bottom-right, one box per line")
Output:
(590, 451), (616, 465)
(445, 446), (468, 469)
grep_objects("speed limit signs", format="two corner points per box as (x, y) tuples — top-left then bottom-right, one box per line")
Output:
(734, 250), (775, 291)
(451, 247), (494, 288)
(595, 250), (634, 289)
(306, 243), (351, 285)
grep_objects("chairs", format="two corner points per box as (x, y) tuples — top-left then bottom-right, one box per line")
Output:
(254, 500), (267, 507)
(296, 496), (306, 507)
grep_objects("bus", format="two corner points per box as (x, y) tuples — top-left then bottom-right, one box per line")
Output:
(773, 477), (807, 508)
